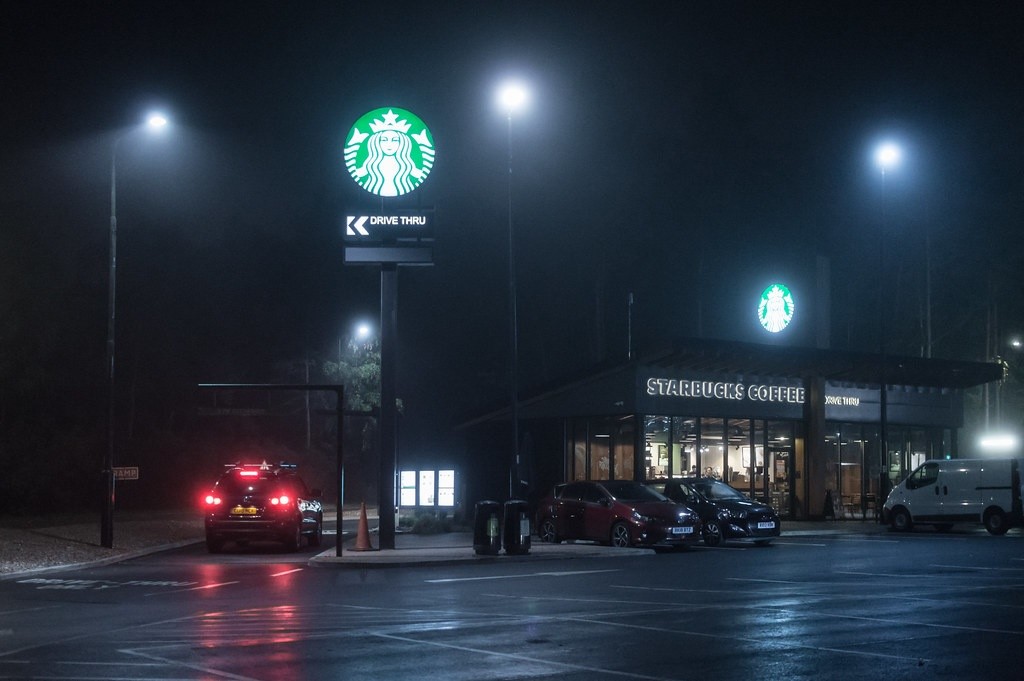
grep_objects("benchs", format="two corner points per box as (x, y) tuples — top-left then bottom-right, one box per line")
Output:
(842, 493), (879, 520)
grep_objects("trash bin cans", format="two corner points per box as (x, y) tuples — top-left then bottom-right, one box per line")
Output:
(503, 500), (532, 556)
(473, 500), (502, 555)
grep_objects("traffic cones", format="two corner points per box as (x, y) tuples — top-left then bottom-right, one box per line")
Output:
(346, 502), (380, 552)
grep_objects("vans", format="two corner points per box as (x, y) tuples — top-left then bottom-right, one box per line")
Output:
(881, 456), (1024, 536)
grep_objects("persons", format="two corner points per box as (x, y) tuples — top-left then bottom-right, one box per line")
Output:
(688, 465), (696, 473)
(702, 466), (717, 480)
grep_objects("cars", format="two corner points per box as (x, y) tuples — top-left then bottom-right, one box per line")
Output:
(531, 476), (703, 552)
(637, 474), (781, 548)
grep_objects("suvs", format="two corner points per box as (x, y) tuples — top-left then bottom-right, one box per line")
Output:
(202, 459), (326, 553)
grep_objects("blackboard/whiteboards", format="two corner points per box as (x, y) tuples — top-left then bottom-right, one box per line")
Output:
(823, 488), (843, 516)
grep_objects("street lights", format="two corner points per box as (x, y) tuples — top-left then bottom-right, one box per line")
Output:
(495, 76), (525, 498)
(875, 143), (902, 524)
(99, 114), (170, 550)
(335, 320), (371, 504)
